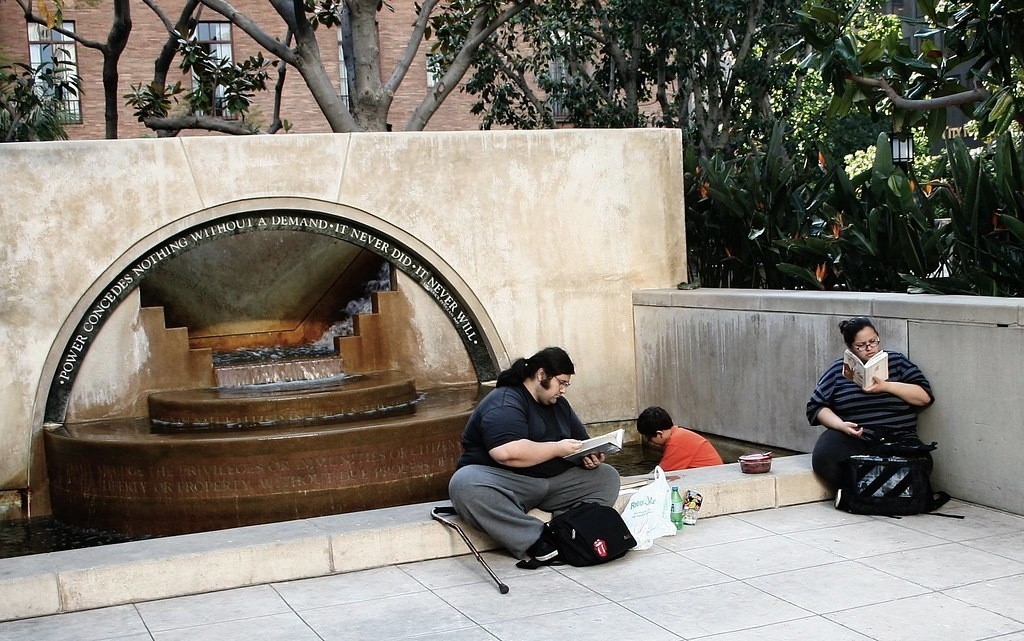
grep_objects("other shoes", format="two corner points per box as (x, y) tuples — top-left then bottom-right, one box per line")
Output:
(527, 539), (558, 562)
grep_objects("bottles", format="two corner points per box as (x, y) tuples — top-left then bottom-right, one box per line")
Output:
(671, 487), (683, 530)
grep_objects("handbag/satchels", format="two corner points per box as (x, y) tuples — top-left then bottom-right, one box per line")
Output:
(618, 466), (677, 551)
(835, 426), (950, 515)
(516, 502), (637, 570)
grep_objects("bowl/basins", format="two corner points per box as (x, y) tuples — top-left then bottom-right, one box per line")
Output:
(740, 455), (771, 472)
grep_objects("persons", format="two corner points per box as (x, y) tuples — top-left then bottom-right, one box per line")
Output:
(806, 318), (935, 511)
(636, 406), (724, 475)
(450, 346), (620, 561)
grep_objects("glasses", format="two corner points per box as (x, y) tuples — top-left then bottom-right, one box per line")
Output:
(554, 375), (570, 388)
(645, 432), (655, 442)
(851, 336), (880, 352)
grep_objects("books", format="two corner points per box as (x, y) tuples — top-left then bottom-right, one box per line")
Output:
(563, 428), (625, 464)
(842, 348), (889, 390)
